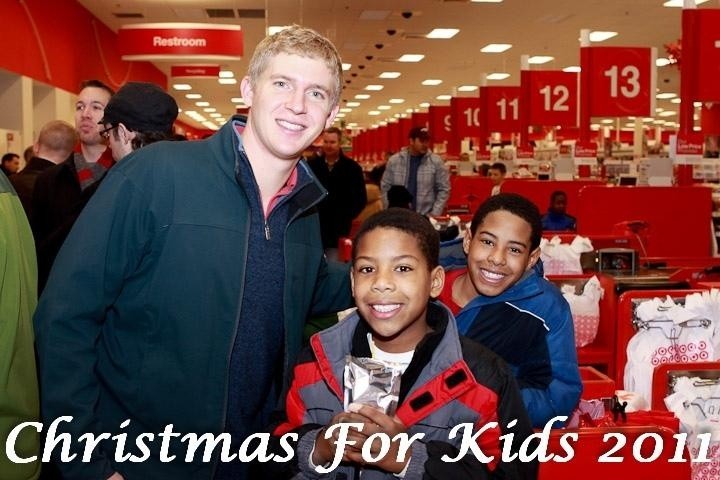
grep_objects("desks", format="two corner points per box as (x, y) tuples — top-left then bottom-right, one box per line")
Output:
(434, 172), (720, 479)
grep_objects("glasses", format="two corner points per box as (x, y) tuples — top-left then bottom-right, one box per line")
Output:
(98, 126), (121, 140)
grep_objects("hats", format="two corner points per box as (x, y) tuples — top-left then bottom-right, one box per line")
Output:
(97, 82), (179, 133)
(408, 126), (431, 141)
